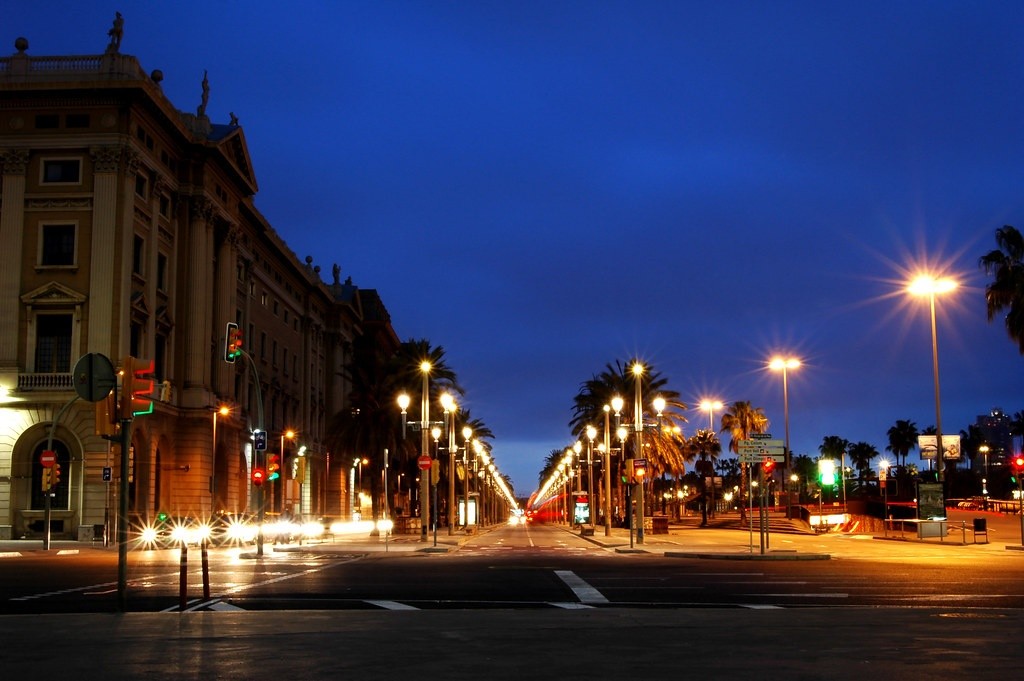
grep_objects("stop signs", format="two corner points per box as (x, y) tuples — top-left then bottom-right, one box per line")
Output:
(40, 450), (56, 467)
(417, 456), (432, 471)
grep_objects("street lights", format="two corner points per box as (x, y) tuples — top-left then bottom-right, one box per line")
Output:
(699, 400), (723, 518)
(534, 404), (627, 527)
(211, 406), (228, 513)
(430, 392), (519, 530)
(280, 431), (296, 516)
(769, 360), (800, 520)
(907, 273), (959, 483)
(611, 364), (664, 544)
(254, 431), (267, 452)
(397, 473), (405, 507)
(396, 360), (453, 541)
(100, 433), (118, 547)
(359, 458), (369, 493)
(878, 460), (889, 537)
(979, 446), (988, 479)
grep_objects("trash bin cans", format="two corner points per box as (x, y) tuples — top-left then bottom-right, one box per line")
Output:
(94, 524), (105, 537)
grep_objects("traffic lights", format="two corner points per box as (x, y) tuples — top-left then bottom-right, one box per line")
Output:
(1016, 459), (1024, 479)
(120, 357), (156, 421)
(52, 462), (60, 484)
(224, 322), (241, 364)
(42, 468), (52, 492)
(266, 454), (279, 482)
(253, 471), (262, 486)
